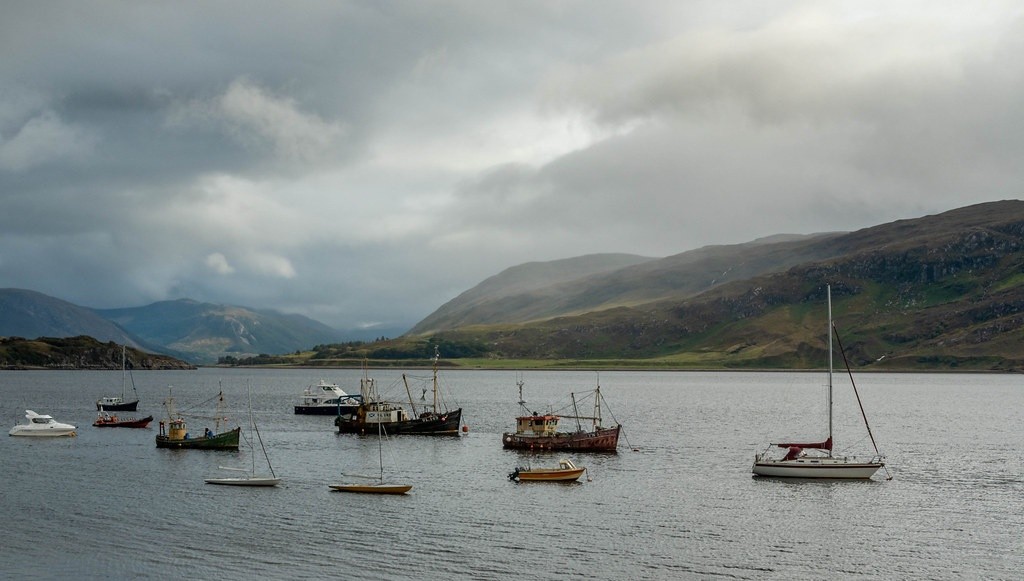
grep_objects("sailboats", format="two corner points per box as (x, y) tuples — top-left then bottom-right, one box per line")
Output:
(203, 376), (281, 486)
(94, 345), (140, 410)
(751, 284), (894, 479)
(327, 380), (413, 495)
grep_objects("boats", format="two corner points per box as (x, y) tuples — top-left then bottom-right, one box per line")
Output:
(334, 345), (465, 439)
(9, 410), (78, 436)
(91, 403), (155, 428)
(508, 458), (589, 483)
(293, 376), (362, 416)
(156, 378), (241, 450)
(501, 371), (625, 454)
